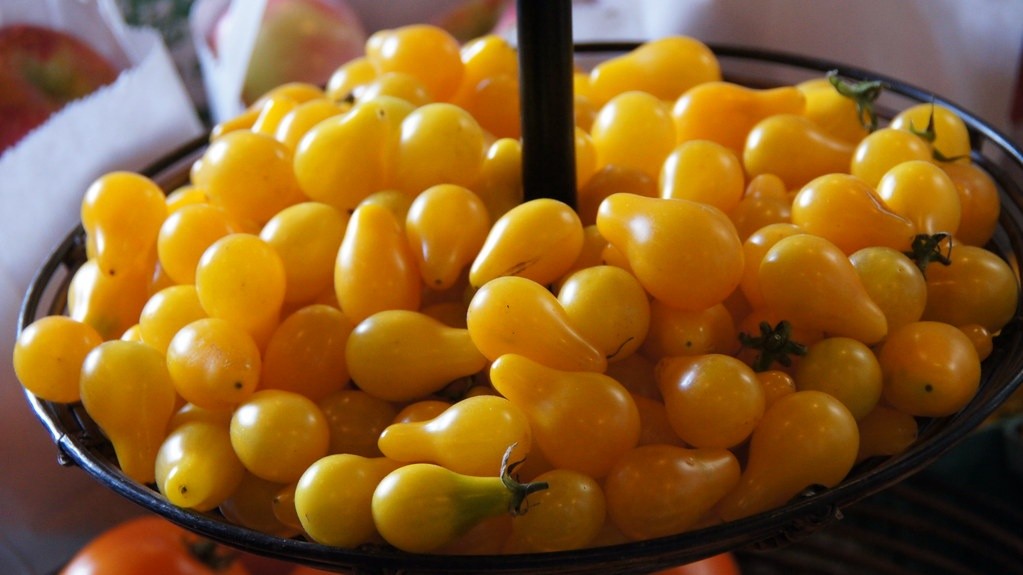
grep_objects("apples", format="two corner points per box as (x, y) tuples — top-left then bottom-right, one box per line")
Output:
(0, 0), (508, 161)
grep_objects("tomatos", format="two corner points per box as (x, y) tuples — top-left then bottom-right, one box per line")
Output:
(12, 25), (1022, 575)
